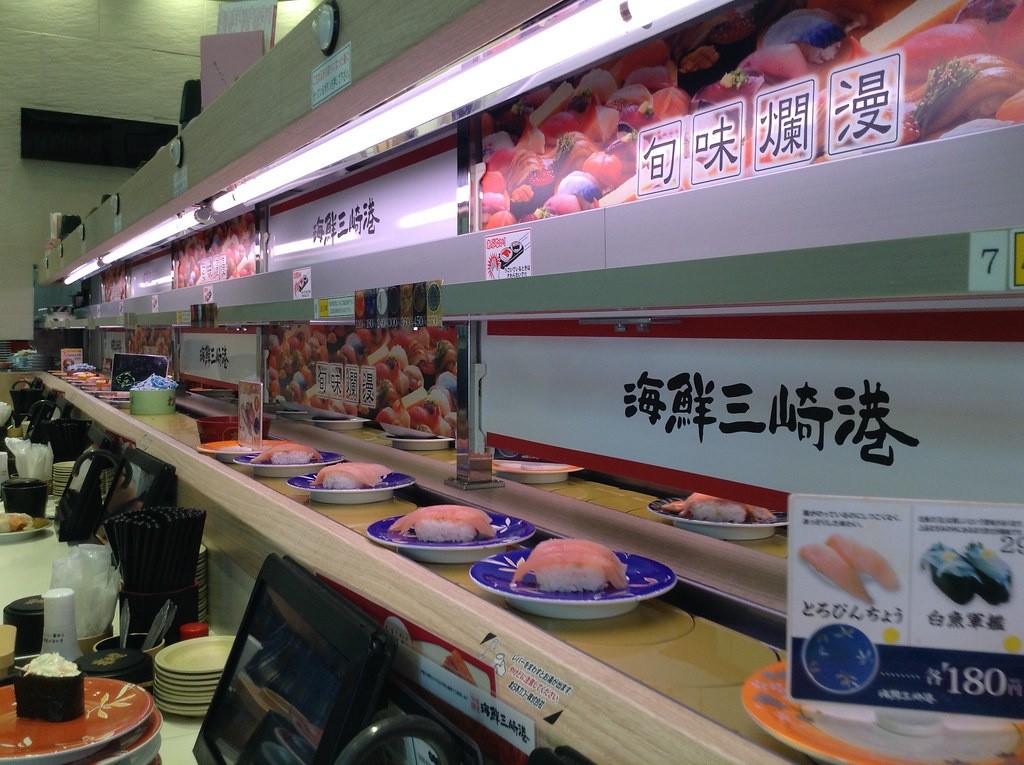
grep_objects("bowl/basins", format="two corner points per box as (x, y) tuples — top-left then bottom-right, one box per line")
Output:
(198, 416), (271, 445)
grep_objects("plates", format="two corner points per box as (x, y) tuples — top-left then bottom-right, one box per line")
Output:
(364, 512), (537, 563)
(197, 439), (293, 464)
(376, 432), (456, 452)
(306, 405), (358, 419)
(492, 458), (585, 483)
(286, 472), (416, 504)
(276, 410), (308, 420)
(304, 416), (371, 429)
(0, 339), (238, 764)
(740, 661), (1023, 765)
(232, 450), (347, 479)
(646, 496), (789, 540)
(381, 421), (440, 439)
(469, 548), (678, 620)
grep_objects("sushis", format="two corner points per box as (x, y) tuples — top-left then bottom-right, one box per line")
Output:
(248, 443), (322, 464)
(386, 505), (497, 542)
(510, 538), (627, 593)
(798, 535), (899, 606)
(920, 540), (1012, 606)
(12, 651), (83, 722)
(661, 491), (776, 524)
(311, 463), (391, 489)
